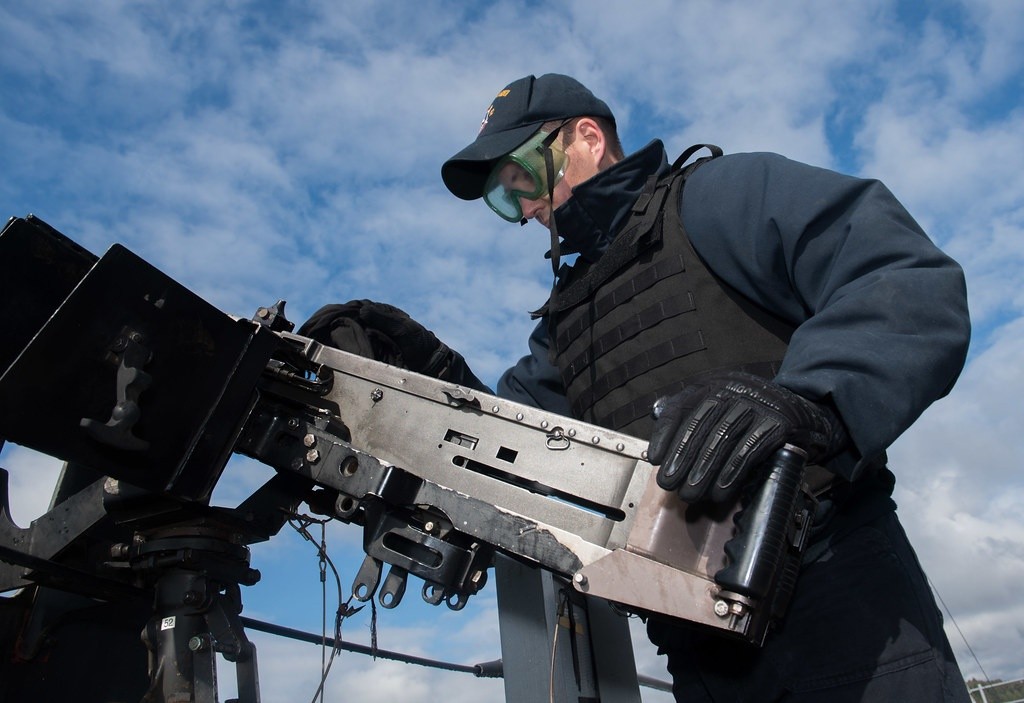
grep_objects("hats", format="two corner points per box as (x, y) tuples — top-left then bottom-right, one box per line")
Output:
(441, 73), (615, 201)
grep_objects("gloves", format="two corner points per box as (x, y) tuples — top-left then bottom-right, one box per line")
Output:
(305, 298), (451, 375)
(647, 370), (849, 508)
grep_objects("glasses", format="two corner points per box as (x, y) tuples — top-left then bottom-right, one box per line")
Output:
(483, 133), (561, 222)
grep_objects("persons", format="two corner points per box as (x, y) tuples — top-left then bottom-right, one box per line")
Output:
(296, 73), (971, 703)
